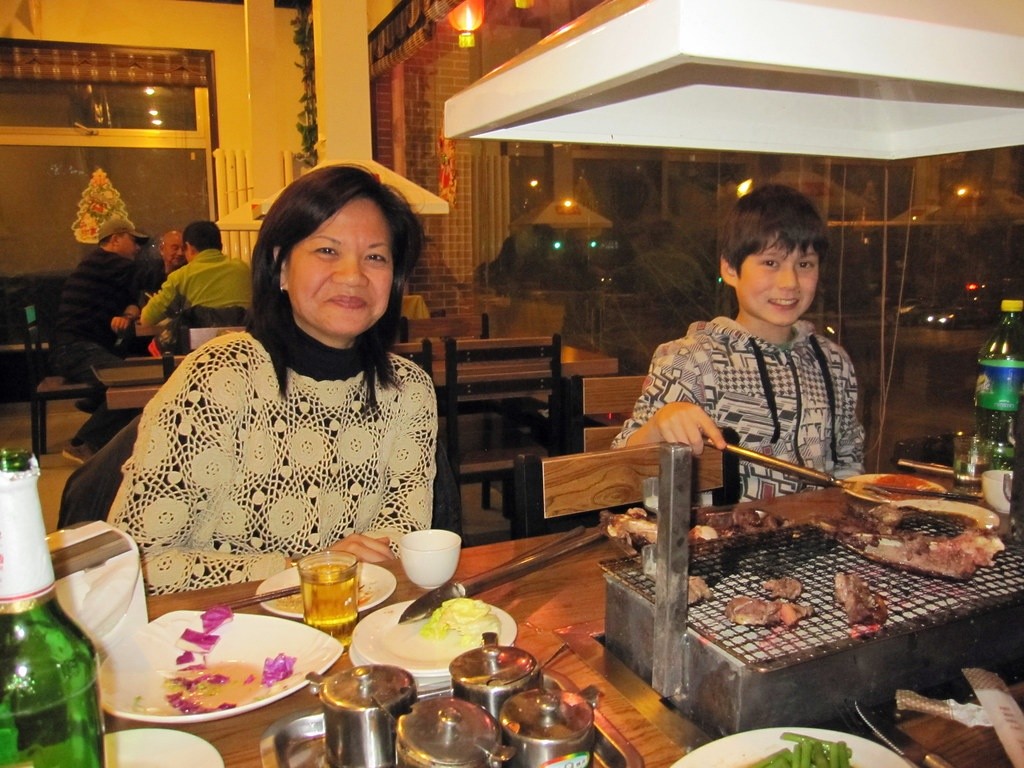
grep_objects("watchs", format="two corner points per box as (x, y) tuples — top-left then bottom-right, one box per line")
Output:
(123, 313), (136, 321)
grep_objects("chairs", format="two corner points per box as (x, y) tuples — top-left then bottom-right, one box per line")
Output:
(25, 301), (727, 545)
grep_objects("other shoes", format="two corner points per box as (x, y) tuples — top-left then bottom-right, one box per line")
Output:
(63, 439), (94, 464)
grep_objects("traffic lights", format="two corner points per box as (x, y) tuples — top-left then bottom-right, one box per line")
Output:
(553, 241), (562, 251)
(589, 241), (598, 249)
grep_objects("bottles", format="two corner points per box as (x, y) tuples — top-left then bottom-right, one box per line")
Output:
(0, 444), (106, 768)
(975, 299), (1024, 470)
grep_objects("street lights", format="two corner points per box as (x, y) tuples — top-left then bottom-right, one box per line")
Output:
(956, 185), (976, 282)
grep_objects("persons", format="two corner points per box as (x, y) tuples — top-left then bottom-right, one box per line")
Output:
(106, 162), (440, 597)
(140, 220), (254, 329)
(49, 218), (150, 464)
(135, 230), (188, 296)
(473, 216), (714, 306)
(609, 181), (866, 513)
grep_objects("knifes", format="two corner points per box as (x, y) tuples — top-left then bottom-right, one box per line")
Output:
(855, 699), (956, 768)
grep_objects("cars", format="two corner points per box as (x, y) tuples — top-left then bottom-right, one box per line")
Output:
(895, 298), (939, 325)
(968, 277), (1024, 301)
(924, 306), (994, 330)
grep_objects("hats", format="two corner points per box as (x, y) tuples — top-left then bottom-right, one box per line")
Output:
(98, 218), (149, 245)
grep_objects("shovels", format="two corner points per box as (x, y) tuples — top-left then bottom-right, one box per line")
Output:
(704, 436), (895, 504)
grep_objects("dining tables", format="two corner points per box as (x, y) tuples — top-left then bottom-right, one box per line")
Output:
(84, 528), (1023, 768)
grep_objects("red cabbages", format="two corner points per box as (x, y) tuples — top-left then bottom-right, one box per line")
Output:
(166, 605), (297, 714)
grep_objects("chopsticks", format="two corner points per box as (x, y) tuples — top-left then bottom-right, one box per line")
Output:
(219, 585), (301, 610)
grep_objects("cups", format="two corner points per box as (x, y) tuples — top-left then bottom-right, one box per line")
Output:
(399, 529), (462, 590)
(954, 436), (994, 498)
(306, 631), (602, 768)
(982, 470), (1013, 513)
(297, 549), (360, 656)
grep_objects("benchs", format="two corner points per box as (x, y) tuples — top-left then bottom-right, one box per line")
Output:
(101, 351), (618, 410)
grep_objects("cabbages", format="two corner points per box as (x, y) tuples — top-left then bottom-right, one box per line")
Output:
(420, 597), (501, 653)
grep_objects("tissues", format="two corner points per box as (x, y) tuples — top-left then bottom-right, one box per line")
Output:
(43, 519), (150, 662)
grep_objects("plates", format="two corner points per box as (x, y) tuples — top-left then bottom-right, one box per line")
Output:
(669, 727), (912, 768)
(843, 473), (947, 504)
(99, 609), (345, 724)
(103, 727), (226, 768)
(255, 561), (397, 618)
(891, 499), (1001, 535)
(348, 598), (518, 693)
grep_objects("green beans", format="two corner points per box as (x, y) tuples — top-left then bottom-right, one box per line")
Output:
(747, 732), (855, 768)
